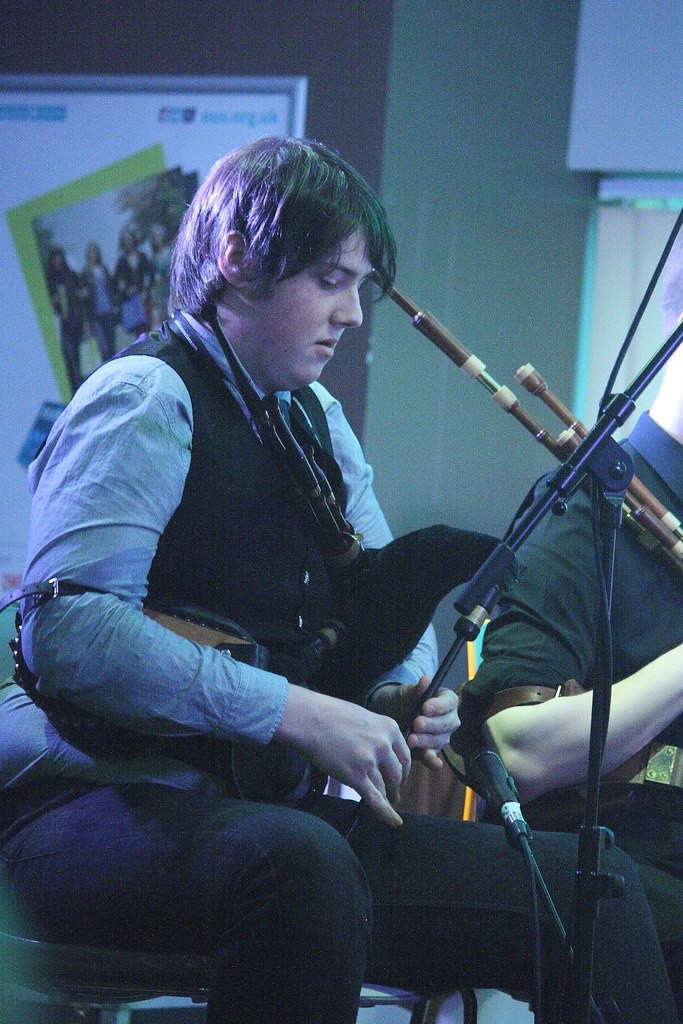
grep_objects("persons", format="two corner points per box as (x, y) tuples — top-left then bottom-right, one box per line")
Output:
(0, 138), (683, 1024)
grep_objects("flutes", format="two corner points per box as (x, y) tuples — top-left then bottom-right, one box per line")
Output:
(371, 267), (683, 582)
(231, 394), (502, 810)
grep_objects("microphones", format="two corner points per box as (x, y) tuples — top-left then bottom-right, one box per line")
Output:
(450, 721), (530, 838)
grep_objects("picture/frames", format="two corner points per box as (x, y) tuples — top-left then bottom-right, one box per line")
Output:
(0, 73), (309, 590)
